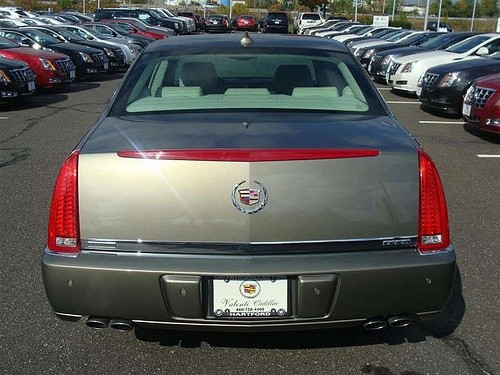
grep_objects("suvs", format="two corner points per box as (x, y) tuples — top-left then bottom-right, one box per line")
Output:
(263, 11), (288, 34)
(427, 21), (455, 33)
(292, 11), (322, 34)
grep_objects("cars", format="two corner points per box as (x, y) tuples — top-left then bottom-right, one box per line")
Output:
(0, 27), (111, 82)
(0, 5), (235, 68)
(41, 34), (463, 334)
(0, 35), (75, 94)
(462, 72), (500, 143)
(368, 32), (486, 84)
(386, 32), (500, 97)
(299, 15), (435, 60)
(417, 50), (500, 118)
(0, 56), (36, 100)
(356, 30), (448, 69)
(235, 14), (257, 31)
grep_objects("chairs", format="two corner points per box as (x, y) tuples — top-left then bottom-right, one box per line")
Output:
(178, 61), (223, 95)
(273, 64), (313, 95)
